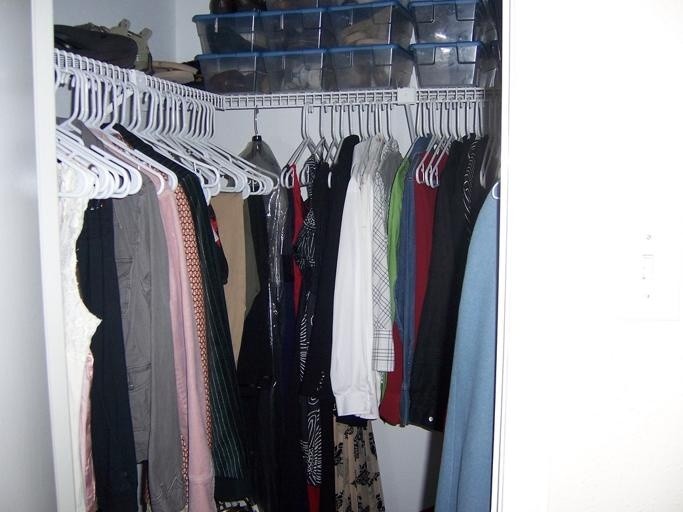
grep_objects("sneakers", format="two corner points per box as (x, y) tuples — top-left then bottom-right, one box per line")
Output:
(408, 1), (501, 42)
(341, 7), (404, 46)
(267, 12), (339, 51)
(415, 47), (498, 87)
(332, 50), (412, 89)
(255, 55), (321, 93)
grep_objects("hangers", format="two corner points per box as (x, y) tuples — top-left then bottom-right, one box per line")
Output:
(430, 96), (457, 189)
(347, 99), (357, 136)
(491, 180), (502, 199)
(414, 97), (430, 144)
(286, 103), (321, 189)
(358, 100), (365, 143)
(475, 95), (501, 189)
(405, 99), (422, 158)
(280, 102), (314, 190)
(386, 98), (394, 150)
(325, 102), (339, 166)
(299, 101), (335, 185)
(334, 103), (346, 164)
(53, 49), (196, 210)
(416, 98), (440, 184)
(367, 101), (374, 138)
(463, 96), (474, 144)
(375, 99), (385, 138)
(454, 97), (464, 146)
(196, 94), (279, 198)
(424, 97), (448, 186)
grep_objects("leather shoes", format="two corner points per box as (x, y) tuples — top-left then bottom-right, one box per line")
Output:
(209, 0), (237, 12)
(208, 70), (253, 92)
(267, 0), (294, 10)
(296, 0), (314, 9)
(238, 0), (266, 12)
(205, 23), (251, 53)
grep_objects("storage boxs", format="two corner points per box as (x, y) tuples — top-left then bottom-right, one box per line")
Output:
(258, 51), (336, 92)
(210, 0), (266, 12)
(409, 0), (501, 43)
(196, 52), (269, 94)
(255, 13), (331, 51)
(324, 4), (413, 46)
(192, 11), (257, 53)
(266, 0), (320, 12)
(328, 45), (413, 91)
(408, 42), (501, 88)
